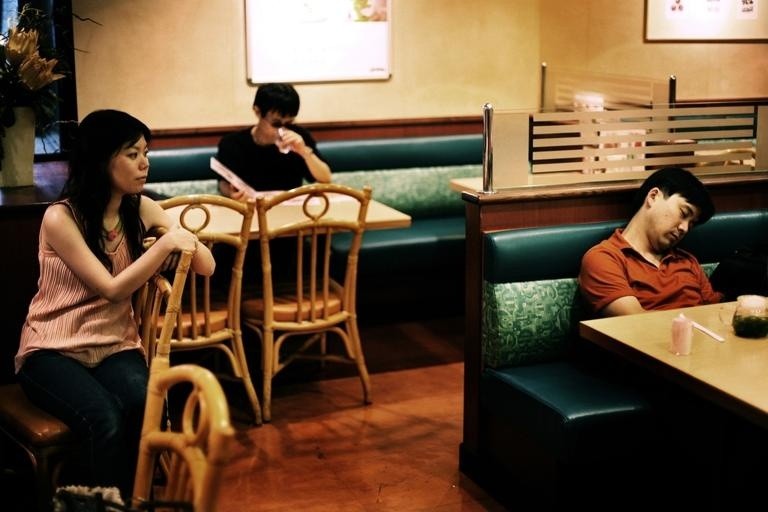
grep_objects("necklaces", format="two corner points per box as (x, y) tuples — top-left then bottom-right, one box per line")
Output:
(98, 220), (123, 241)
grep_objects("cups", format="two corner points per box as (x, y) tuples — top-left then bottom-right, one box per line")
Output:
(672, 318), (691, 355)
(272, 126), (291, 154)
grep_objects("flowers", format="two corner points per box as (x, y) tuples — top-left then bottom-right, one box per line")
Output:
(0, 0), (104, 124)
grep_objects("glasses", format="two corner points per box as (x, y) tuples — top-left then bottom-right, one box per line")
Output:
(260, 113), (293, 129)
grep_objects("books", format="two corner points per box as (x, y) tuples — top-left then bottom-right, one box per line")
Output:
(209, 150), (323, 206)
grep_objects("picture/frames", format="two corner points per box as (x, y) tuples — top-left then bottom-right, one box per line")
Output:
(645, 0), (768, 42)
(243, 1), (394, 86)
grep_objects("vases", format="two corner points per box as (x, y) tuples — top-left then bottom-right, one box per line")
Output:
(0, 105), (37, 187)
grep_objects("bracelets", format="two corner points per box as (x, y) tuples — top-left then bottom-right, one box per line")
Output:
(301, 146), (314, 158)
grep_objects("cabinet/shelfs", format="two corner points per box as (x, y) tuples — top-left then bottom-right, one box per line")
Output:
(0, 183), (54, 351)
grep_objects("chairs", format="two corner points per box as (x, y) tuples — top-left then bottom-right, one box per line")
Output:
(132, 360), (236, 511)
(723, 151), (756, 170)
(240, 183), (373, 422)
(134, 196), (262, 432)
(0, 233), (196, 476)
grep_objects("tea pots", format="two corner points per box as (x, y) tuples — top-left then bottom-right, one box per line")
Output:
(719, 294), (768, 339)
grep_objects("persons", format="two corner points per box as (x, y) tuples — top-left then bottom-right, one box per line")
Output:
(577, 166), (725, 511)
(13, 110), (215, 512)
(209, 85), (333, 396)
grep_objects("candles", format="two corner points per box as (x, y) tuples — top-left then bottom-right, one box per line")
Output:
(672, 312), (693, 355)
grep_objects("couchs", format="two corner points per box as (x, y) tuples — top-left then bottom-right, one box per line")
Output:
(478, 209), (768, 511)
(144, 128), (487, 277)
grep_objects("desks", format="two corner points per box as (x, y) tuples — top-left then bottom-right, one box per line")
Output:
(145, 185), (412, 249)
(449, 172), (484, 195)
(580, 297), (767, 423)
